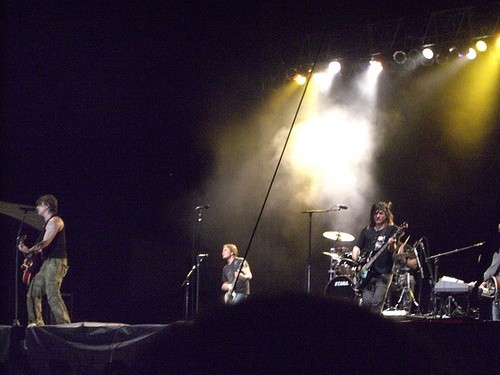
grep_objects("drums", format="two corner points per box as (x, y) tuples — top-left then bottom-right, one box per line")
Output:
(324, 275), (362, 305)
(332, 258), (362, 289)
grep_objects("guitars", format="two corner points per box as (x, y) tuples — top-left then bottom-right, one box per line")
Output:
(225, 264), (244, 305)
(18, 235), (33, 285)
(352, 223), (409, 291)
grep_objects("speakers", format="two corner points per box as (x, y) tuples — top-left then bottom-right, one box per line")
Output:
(42, 292), (73, 325)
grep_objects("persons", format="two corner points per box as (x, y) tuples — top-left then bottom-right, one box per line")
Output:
(387, 225), (417, 312)
(352, 202), (399, 315)
(19, 195), (71, 326)
(480, 224), (500, 321)
(221, 244), (252, 305)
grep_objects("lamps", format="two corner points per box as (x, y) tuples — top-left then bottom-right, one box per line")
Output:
(286, 36), (494, 85)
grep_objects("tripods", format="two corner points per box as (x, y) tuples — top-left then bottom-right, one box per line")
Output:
(377, 246), (464, 319)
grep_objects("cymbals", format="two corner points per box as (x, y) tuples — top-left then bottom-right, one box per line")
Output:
(322, 230), (356, 242)
(320, 250), (343, 260)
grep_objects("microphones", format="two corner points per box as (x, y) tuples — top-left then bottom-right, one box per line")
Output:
(198, 253), (208, 257)
(195, 204), (209, 210)
(434, 257), (440, 266)
(19, 207), (36, 211)
(473, 241), (486, 246)
(337, 205), (348, 209)
